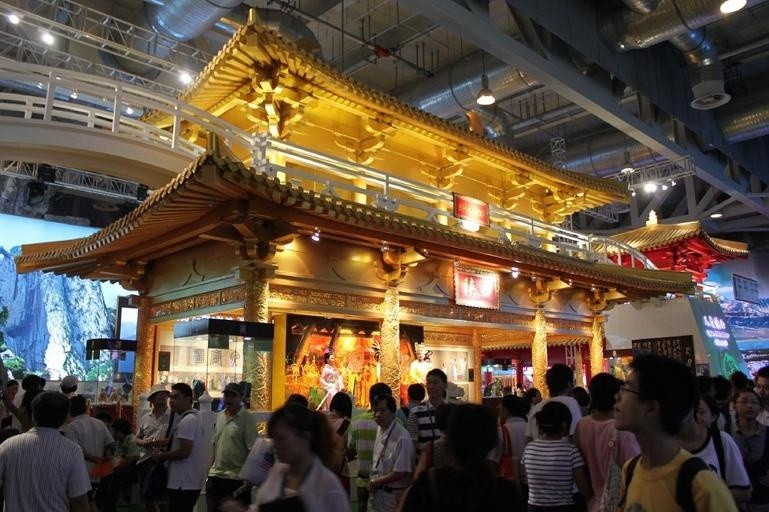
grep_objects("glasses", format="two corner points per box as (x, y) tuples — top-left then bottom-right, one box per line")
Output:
(619, 385), (637, 396)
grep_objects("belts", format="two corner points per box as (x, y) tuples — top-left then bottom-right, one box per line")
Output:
(382, 485), (405, 494)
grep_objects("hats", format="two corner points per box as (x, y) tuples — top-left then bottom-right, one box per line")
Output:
(147, 384), (170, 402)
(22, 375), (46, 389)
(222, 383), (242, 395)
(446, 383), (465, 398)
(60, 376), (78, 388)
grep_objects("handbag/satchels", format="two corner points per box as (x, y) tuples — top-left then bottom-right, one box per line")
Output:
(500, 452), (513, 479)
(239, 439), (275, 487)
(600, 457), (622, 511)
(136, 455), (169, 502)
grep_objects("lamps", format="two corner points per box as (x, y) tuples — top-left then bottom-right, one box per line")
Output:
(311, 229), (321, 242)
(476, 51), (495, 105)
(621, 136), (634, 173)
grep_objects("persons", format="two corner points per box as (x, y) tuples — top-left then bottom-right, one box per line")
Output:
(2, 347), (767, 510)
